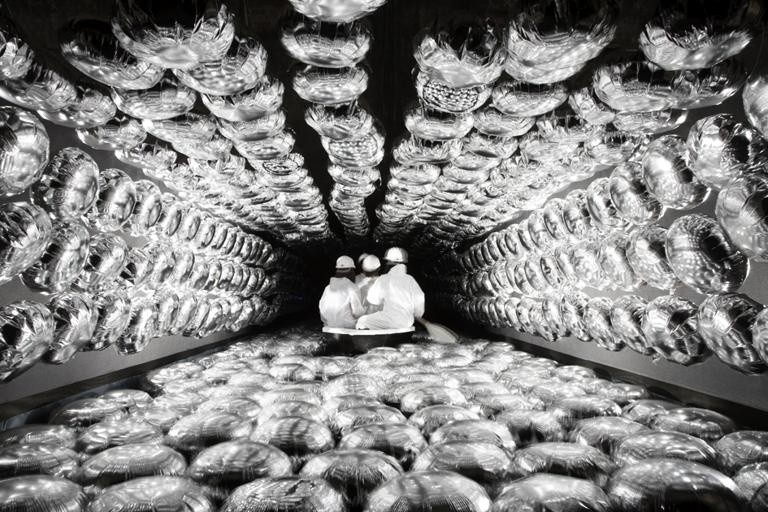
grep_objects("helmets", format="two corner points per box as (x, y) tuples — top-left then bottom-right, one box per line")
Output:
(335, 247), (408, 273)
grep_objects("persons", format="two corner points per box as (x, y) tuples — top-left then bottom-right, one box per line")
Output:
(354, 253), (369, 282)
(355, 247), (426, 330)
(357, 254), (382, 307)
(318, 255), (365, 329)
